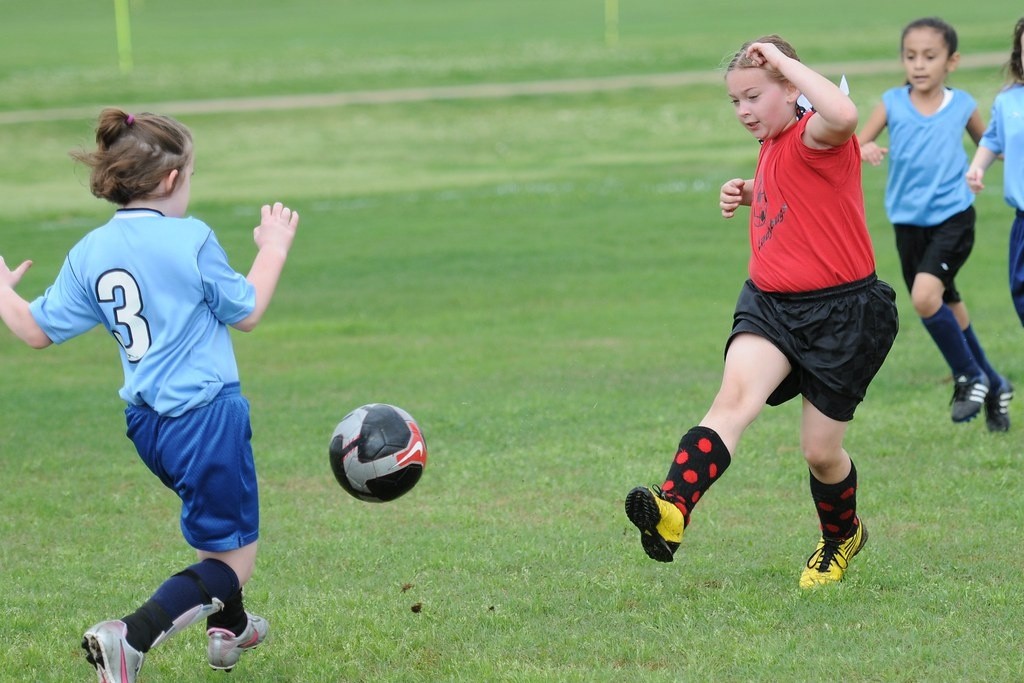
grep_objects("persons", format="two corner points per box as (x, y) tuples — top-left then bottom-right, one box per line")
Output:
(625, 34), (900, 589)
(857, 16), (1015, 432)
(966, 16), (1024, 327)
(0, 108), (299, 683)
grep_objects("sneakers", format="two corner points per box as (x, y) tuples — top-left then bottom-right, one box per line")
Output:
(985, 378), (1015, 432)
(949, 374), (992, 422)
(81, 620), (143, 683)
(625, 484), (684, 563)
(799, 515), (869, 589)
(205, 611), (268, 672)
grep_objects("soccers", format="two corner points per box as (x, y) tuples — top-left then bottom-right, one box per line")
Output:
(329, 403), (427, 504)
